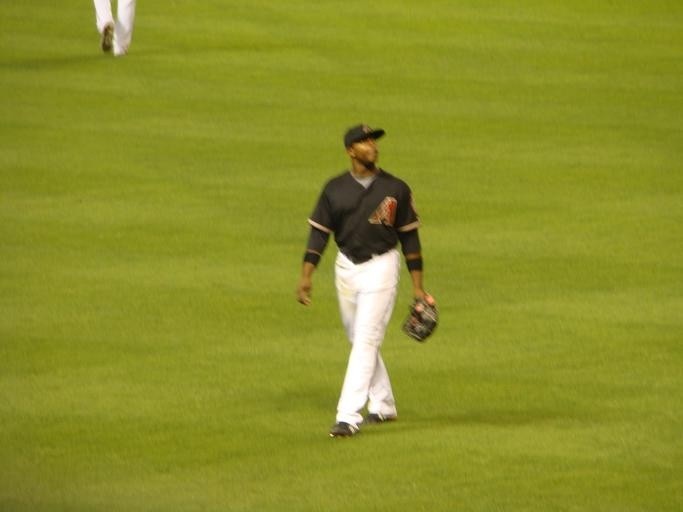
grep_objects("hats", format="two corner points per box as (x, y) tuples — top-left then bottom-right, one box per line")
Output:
(344, 125), (384, 147)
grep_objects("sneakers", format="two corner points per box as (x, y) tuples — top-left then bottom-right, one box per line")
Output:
(102, 24), (113, 50)
(328, 422), (361, 439)
(362, 414), (399, 425)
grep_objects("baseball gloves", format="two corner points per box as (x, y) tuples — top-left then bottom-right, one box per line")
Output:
(402, 296), (438, 342)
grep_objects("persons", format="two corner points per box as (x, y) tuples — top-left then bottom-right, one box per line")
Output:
(90, 0), (138, 58)
(296, 124), (439, 437)
